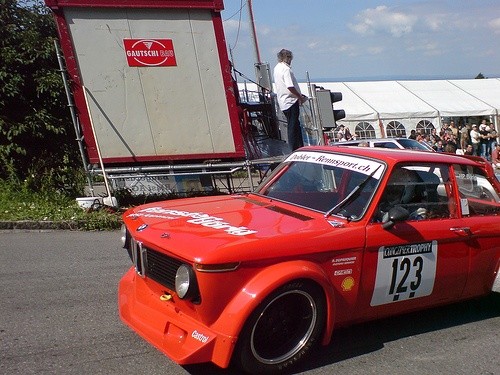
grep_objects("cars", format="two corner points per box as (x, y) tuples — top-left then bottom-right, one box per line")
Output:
(117, 147), (500, 373)
(332, 136), (434, 154)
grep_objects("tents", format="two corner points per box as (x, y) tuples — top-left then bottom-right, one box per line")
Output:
(237, 77), (500, 142)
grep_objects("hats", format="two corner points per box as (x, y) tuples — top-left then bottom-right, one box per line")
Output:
(339, 124), (345, 126)
(471, 124), (476, 127)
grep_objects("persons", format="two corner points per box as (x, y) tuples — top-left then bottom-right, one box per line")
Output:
(273, 49), (308, 152)
(228, 59), (240, 105)
(323, 119), (500, 175)
(376, 168), (428, 222)
